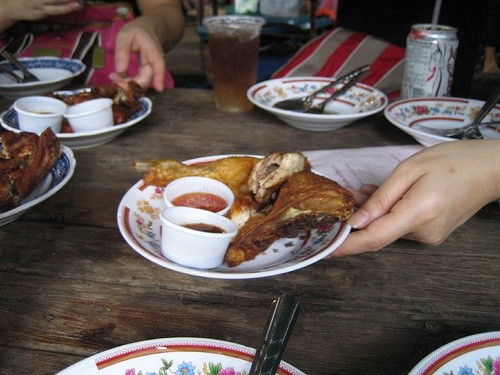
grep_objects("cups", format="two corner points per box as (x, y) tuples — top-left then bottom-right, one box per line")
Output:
(13, 94), (67, 135)
(164, 176), (235, 217)
(202, 15), (268, 111)
(63, 97), (115, 133)
(158, 206), (238, 268)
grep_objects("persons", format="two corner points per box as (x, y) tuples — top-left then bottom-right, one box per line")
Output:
(322, 140), (500, 262)
(332, 0), (500, 72)
(0, 0), (185, 94)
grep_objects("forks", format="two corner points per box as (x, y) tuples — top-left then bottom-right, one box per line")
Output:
(421, 120), (499, 137)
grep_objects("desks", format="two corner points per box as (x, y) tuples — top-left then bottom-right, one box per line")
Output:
(0, 89), (500, 375)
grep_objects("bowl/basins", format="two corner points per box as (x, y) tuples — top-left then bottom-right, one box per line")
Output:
(0, 56), (86, 99)
(0, 86), (152, 150)
(247, 76), (388, 131)
(383, 96), (500, 148)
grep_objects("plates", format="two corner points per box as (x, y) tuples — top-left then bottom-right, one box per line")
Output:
(117, 154), (355, 280)
(56, 337), (306, 375)
(407, 331), (500, 375)
(0, 142), (76, 226)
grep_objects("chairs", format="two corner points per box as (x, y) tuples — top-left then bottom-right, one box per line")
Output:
(197, 0), (331, 77)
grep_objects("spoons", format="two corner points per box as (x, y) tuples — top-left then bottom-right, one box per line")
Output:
(461, 88), (500, 140)
(272, 64), (372, 112)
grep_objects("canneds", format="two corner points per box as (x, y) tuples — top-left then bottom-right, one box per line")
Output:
(400, 23), (457, 97)
